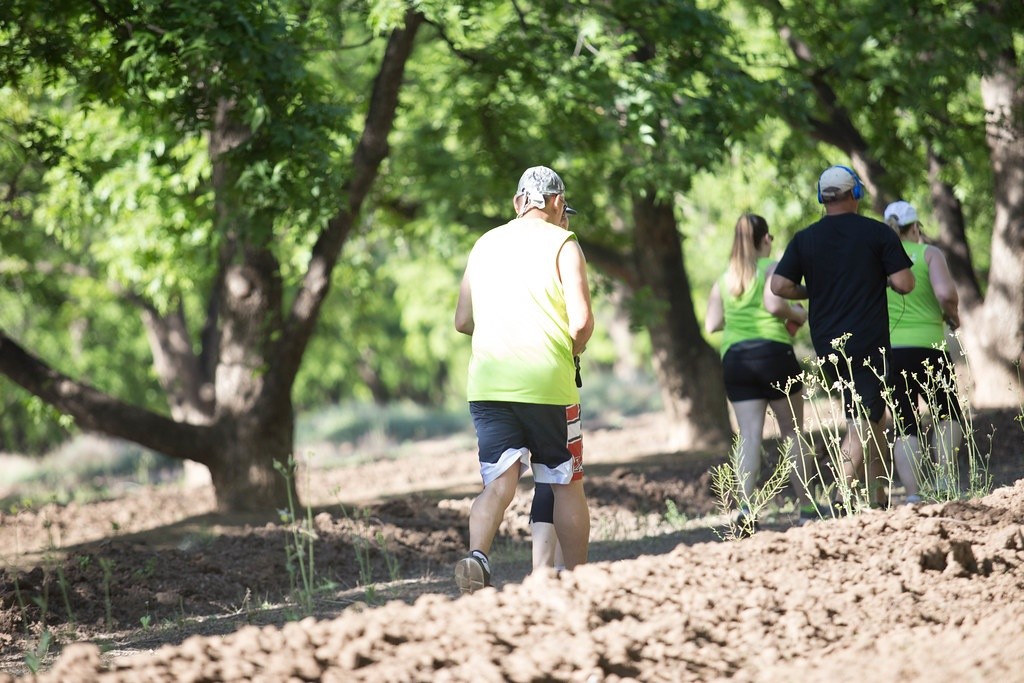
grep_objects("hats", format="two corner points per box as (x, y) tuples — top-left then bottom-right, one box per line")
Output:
(563, 201), (578, 215)
(513, 166), (565, 219)
(884, 201), (923, 228)
(819, 165), (864, 197)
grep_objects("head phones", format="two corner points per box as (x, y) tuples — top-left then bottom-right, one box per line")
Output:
(818, 165), (864, 203)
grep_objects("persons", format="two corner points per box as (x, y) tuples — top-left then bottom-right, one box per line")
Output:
(883, 199), (963, 506)
(705, 211), (825, 537)
(768, 164), (918, 520)
(453, 164), (596, 596)
(527, 199), (584, 572)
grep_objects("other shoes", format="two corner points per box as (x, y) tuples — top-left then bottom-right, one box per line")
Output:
(735, 502), (761, 536)
(831, 501), (856, 518)
(454, 550), (491, 595)
(870, 497), (892, 510)
(799, 502), (826, 525)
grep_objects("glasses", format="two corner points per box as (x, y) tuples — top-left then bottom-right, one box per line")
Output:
(769, 235), (773, 241)
